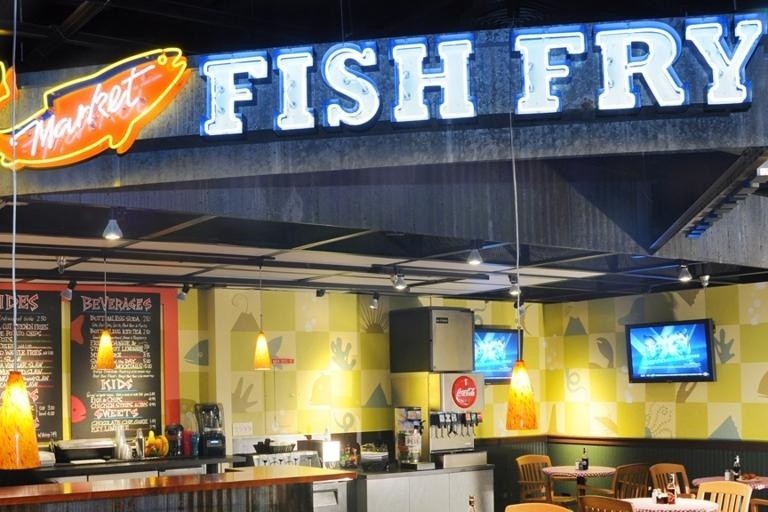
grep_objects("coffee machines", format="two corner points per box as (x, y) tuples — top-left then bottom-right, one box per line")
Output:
(194, 401), (227, 459)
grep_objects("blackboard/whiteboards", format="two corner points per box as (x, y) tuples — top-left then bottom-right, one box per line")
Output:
(1, 281), (178, 449)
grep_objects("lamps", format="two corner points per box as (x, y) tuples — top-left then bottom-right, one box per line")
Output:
(369, 292), (381, 310)
(93, 255), (115, 371)
(393, 272), (408, 289)
(176, 284), (191, 301)
(253, 258), (275, 370)
(756, 160), (768, 176)
(507, 274), (522, 295)
(60, 283), (78, 302)
(676, 258), (693, 282)
(102, 207), (127, 241)
(0, 0), (43, 470)
(465, 240), (484, 266)
(503, 0), (539, 432)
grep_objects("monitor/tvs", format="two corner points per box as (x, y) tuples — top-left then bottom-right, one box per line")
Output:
(625, 318), (716, 383)
(472, 328), (523, 385)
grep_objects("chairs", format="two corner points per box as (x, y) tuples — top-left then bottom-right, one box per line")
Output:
(504, 454), (768, 512)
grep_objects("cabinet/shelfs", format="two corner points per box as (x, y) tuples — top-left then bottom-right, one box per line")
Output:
(350, 463), (495, 512)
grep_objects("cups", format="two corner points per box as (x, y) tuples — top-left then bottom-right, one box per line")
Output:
(108, 423), (132, 462)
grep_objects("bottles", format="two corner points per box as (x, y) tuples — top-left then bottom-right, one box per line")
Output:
(665, 471), (676, 504)
(580, 447), (589, 470)
(341, 443), (360, 465)
(731, 454), (745, 481)
(134, 425), (200, 459)
